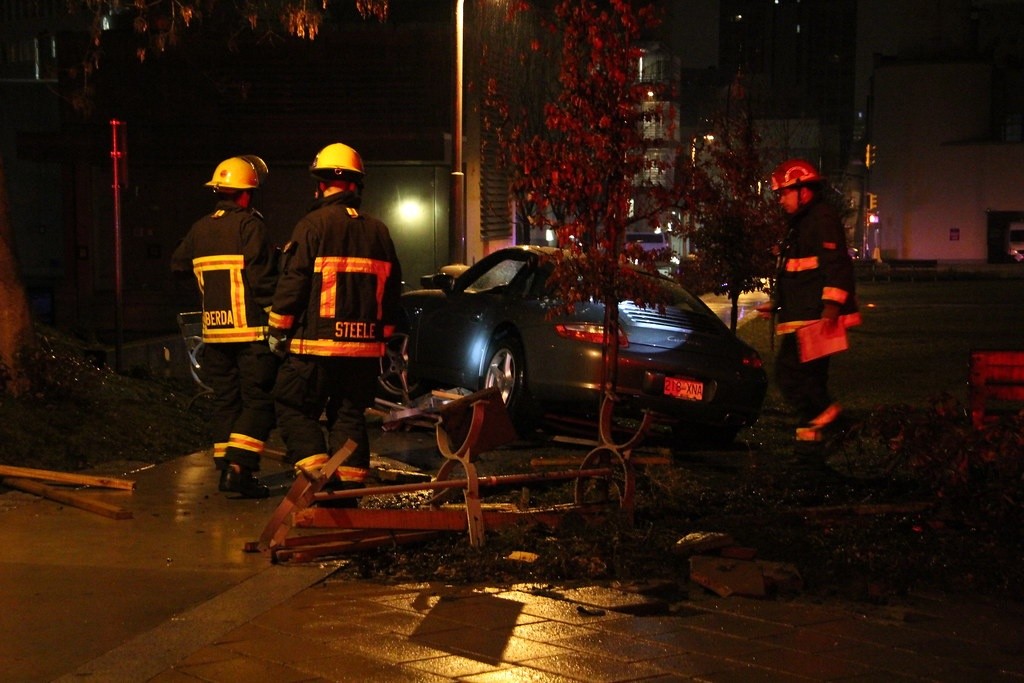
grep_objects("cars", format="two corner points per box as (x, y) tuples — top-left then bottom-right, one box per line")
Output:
(373, 246), (769, 455)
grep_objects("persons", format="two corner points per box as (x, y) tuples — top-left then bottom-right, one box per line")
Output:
(769, 157), (857, 454)
(174, 154), (278, 499)
(267, 140), (402, 509)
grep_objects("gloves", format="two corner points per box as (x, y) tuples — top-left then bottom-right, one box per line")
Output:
(268, 335), (286, 357)
(821, 300), (840, 326)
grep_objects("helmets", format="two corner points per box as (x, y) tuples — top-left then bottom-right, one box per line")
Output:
(772, 161), (825, 191)
(312, 143), (365, 179)
(205, 158), (259, 189)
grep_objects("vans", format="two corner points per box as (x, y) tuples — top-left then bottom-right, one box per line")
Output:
(1001, 220), (1024, 262)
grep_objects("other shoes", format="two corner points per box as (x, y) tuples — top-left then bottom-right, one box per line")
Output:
(219, 459), (270, 498)
(794, 439), (836, 458)
(313, 482), (365, 509)
